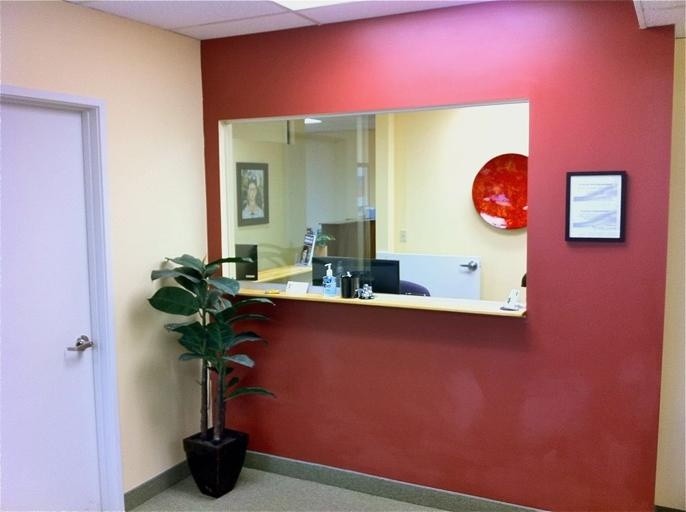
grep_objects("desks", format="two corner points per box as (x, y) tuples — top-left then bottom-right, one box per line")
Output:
(237, 219), (375, 293)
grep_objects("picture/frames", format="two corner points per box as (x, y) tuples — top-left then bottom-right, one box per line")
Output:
(236, 162), (270, 227)
(564, 170), (627, 243)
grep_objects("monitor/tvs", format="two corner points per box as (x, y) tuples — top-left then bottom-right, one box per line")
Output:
(312, 257), (399, 294)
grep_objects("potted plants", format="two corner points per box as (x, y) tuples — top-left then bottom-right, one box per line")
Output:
(147, 253), (277, 498)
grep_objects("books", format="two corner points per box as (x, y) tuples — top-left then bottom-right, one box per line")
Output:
(299, 233), (316, 266)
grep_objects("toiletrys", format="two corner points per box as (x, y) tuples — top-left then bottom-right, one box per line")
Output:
(322, 263), (337, 298)
(340, 271), (360, 299)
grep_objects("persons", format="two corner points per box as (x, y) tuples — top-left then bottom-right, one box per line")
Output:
(300, 246), (309, 265)
(241, 180), (265, 220)
